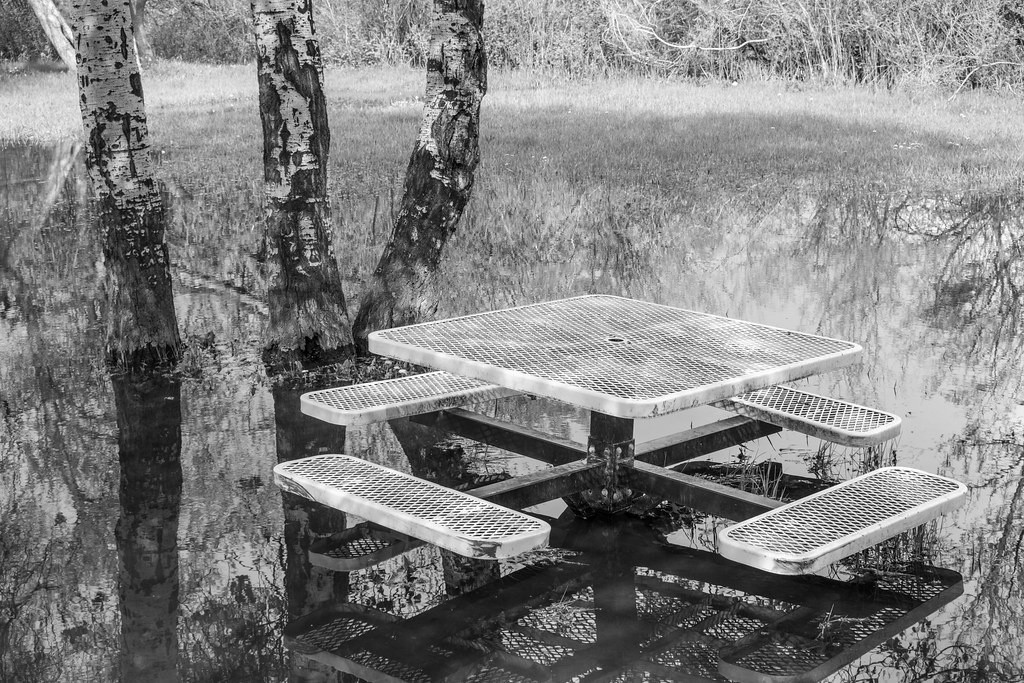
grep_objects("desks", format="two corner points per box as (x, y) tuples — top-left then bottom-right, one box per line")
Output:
(337, 563), (854, 683)
(369, 294), (863, 520)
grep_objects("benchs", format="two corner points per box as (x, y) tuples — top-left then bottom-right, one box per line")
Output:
(300, 370), (527, 426)
(707, 386), (902, 448)
(716, 561), (964, 683)
(717, 466), (968, 575)
(667, 460), (842, 502)
(308, 473), (514, 572)
(272, 454), (552, 560)
(282, 603), (553, 683)
